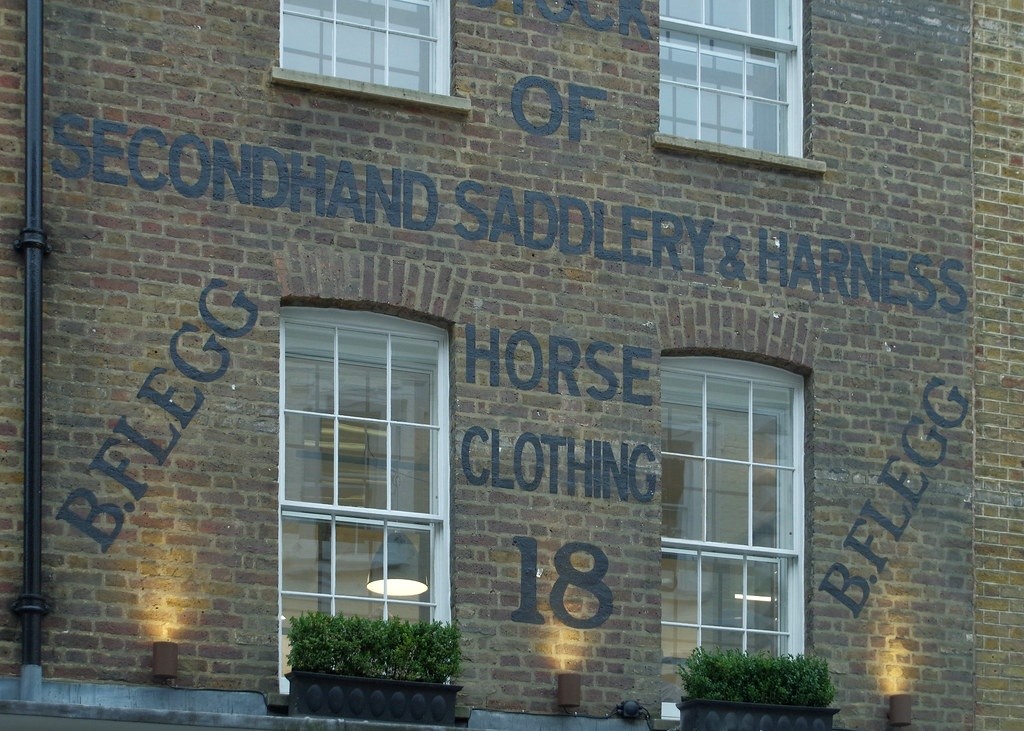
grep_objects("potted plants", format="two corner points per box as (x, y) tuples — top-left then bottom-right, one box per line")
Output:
(677, 647), (841, 730)
(282, 613), (462, 727)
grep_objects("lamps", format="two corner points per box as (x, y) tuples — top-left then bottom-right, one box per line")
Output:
(367, 473), (429, 597)
(733, 561), (777, 603)
(556, 670), (581, 708)
(887, 694), (912, 725)
(151, 642), (179, 679)
